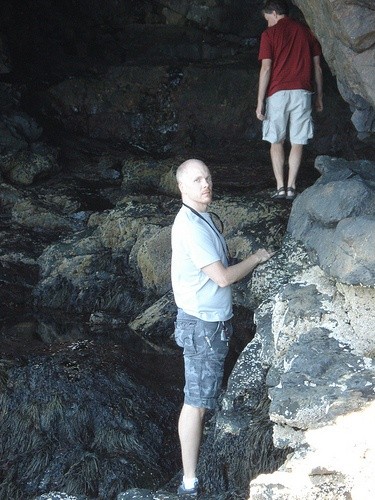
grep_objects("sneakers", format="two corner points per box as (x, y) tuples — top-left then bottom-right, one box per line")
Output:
(177, 481), (204, 500)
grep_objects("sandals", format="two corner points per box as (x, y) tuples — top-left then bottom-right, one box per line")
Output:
(284, 187), (297, 199)
(269, 187), (286, 198)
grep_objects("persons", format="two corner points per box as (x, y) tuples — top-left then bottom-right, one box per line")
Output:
(170, 157), (272, 500)
(256, 0), (324, 200)
